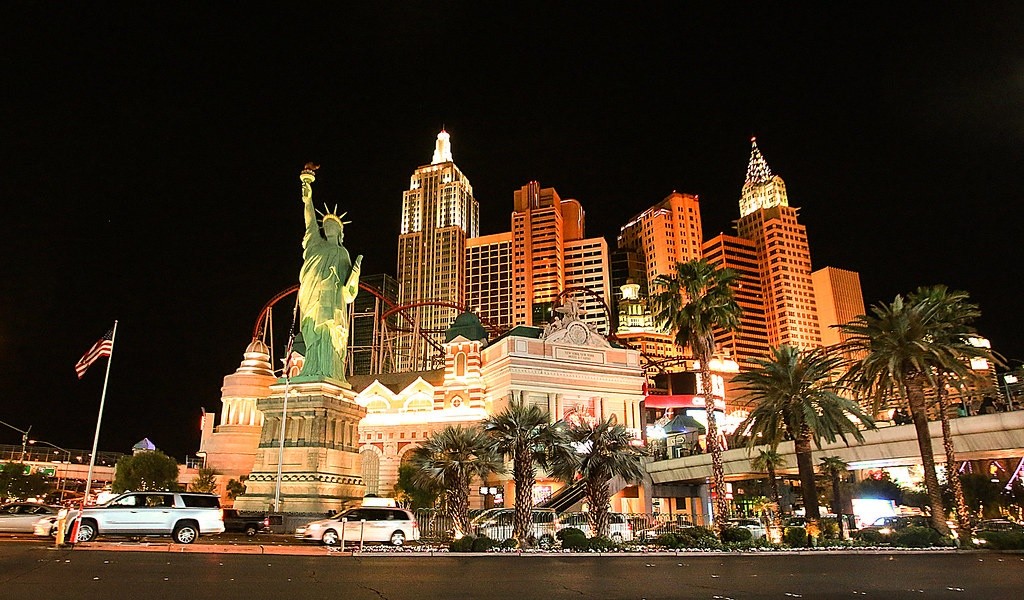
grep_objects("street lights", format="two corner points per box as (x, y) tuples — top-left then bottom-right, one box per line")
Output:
(29, 440), (71, 501)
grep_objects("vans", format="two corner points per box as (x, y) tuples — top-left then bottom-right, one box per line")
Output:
(782, 517), (847, 542)
(454, 507), (562, 551)
(294, 498), (421, 545)
(556, 511), (635, 545)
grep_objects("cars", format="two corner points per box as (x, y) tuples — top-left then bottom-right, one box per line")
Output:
(34, 516), (58, 538)
(859, 516), (907, 542)
(720, 519), (776, 543)
(634, 520), (715, 543)
(5, 489), (82, 505)
(973, 519), (1024, 536)
(0, 502), (58, 533)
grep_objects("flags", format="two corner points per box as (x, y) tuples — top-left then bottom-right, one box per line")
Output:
(75, 330), (114, 379)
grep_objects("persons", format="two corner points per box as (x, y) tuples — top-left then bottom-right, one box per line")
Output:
(967, 396), (973, 416)
(893, 407), (912, 426)
(1011, 396), (1020, 410)
(297, 182), (363, 383)
(983, 393), (996, 412)
(542, 298), (598, 339)
(995, 397), (1006, 411)
(121, 496), (146, 507)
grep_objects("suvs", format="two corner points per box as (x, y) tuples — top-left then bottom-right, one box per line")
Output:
(51, 491), (226, 544)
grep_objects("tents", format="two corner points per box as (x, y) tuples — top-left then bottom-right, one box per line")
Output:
(662, 415), (706, 434)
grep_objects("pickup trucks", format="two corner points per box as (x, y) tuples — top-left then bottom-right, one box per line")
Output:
(223, 509), (269, 537)
